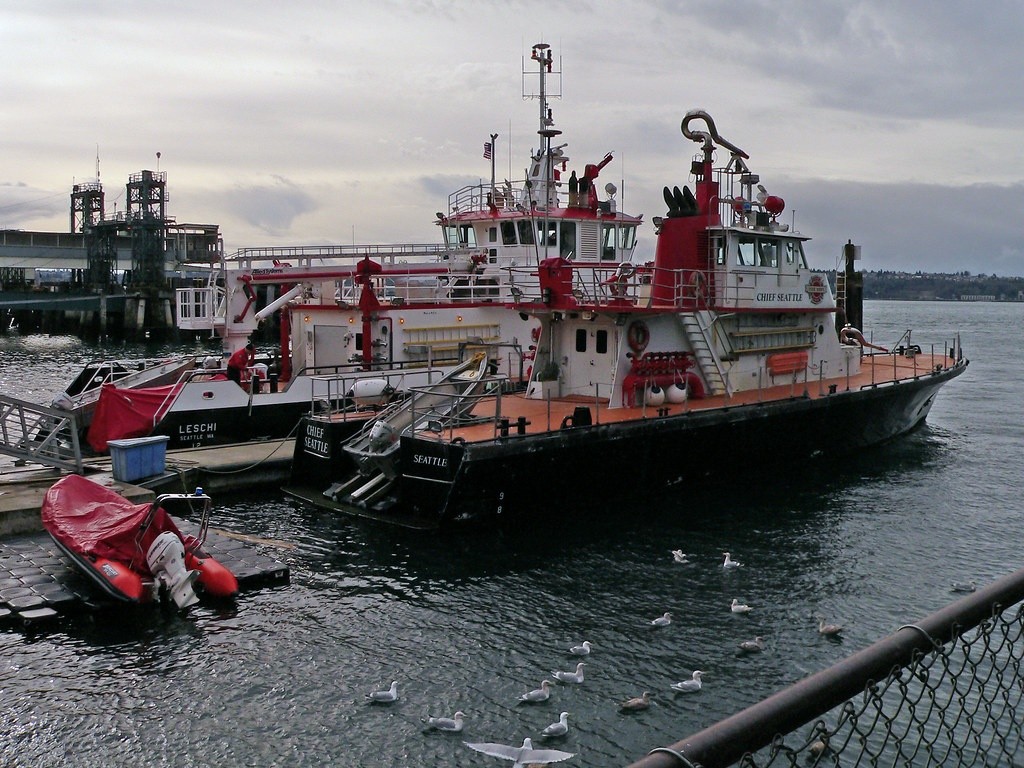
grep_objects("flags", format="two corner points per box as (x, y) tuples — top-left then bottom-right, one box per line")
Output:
(483, 141), (491, 159)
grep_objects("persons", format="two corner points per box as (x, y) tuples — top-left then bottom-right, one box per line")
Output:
(226, 344), (254, 388)
(569, 170), (578, 206)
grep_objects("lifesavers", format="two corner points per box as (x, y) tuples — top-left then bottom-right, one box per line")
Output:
(610, 272), (628, 295)
(688, 270), (707, 296)
(628, 321), (650, 351)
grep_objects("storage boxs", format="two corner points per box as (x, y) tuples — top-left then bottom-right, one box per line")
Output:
(107, 436), (171, 483)
(531, 380), (560, 400)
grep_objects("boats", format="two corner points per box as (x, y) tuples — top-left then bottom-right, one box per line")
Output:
(279, 105), (970, 540)
(31, 33), (655, 460)
(40, 472), (240, 607)
